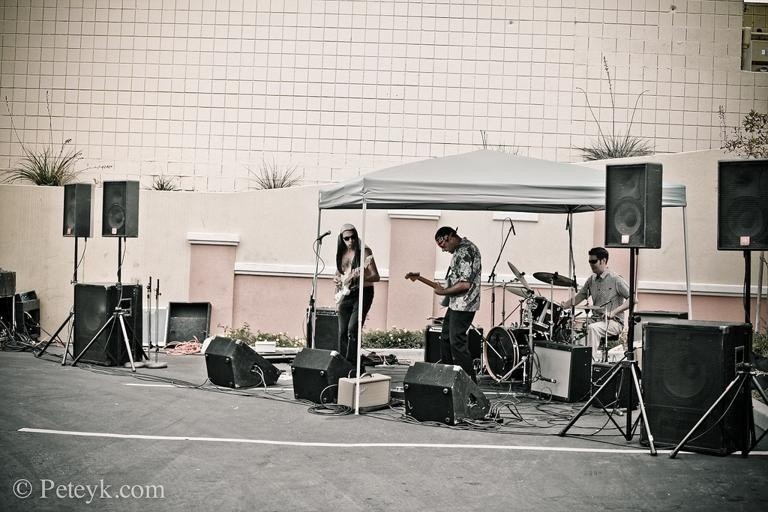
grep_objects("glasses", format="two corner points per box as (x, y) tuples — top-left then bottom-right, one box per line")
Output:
(589, 259), (600, 264)
(343, 235), (355, 241)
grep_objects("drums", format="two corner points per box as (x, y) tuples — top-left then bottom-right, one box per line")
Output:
(524, 296), (566, 335)
(483, 327), (549, 383)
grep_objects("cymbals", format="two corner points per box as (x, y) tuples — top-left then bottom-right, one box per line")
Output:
(574, 305), (606, 309)
(508, 262), (535, 292)
(533, 272), (579, 287)
(505, 286), (537, 299)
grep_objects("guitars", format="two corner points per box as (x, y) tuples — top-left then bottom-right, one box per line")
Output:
(405, 272), (452, 306)
(335, 255), (372, 304)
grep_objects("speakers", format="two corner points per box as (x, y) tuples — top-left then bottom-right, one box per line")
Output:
(205, 336), (281, 388)
(717, 159), (768, 251)
(102, 181), (139, 237)
(641, 317), (752, 456)
(72, 284), (143, 366)
(63, 183), (91, 236)
(291, 347), (355, 402)
(604, 163), (663, 248)
(403, 361), (491, 425)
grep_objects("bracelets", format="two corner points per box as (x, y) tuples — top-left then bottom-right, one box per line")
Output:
(443, 288), (448, 294)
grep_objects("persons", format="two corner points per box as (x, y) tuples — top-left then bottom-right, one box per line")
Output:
(333, 223), (379, 363)
(560, 247), (638, 382)
(434, 227), (481, 403)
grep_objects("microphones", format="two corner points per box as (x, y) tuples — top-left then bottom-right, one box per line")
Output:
(316, 230), (331, 243)
(508, 218), (517, 236)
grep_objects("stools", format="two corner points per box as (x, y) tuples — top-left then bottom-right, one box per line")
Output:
(600, 336), (619, 361)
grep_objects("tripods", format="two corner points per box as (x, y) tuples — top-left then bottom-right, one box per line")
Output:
(34, 238), (90, 367)
(71, 238), (148, 373)
(555, 248), (659, 456)
(667, 251), (768, 460)
